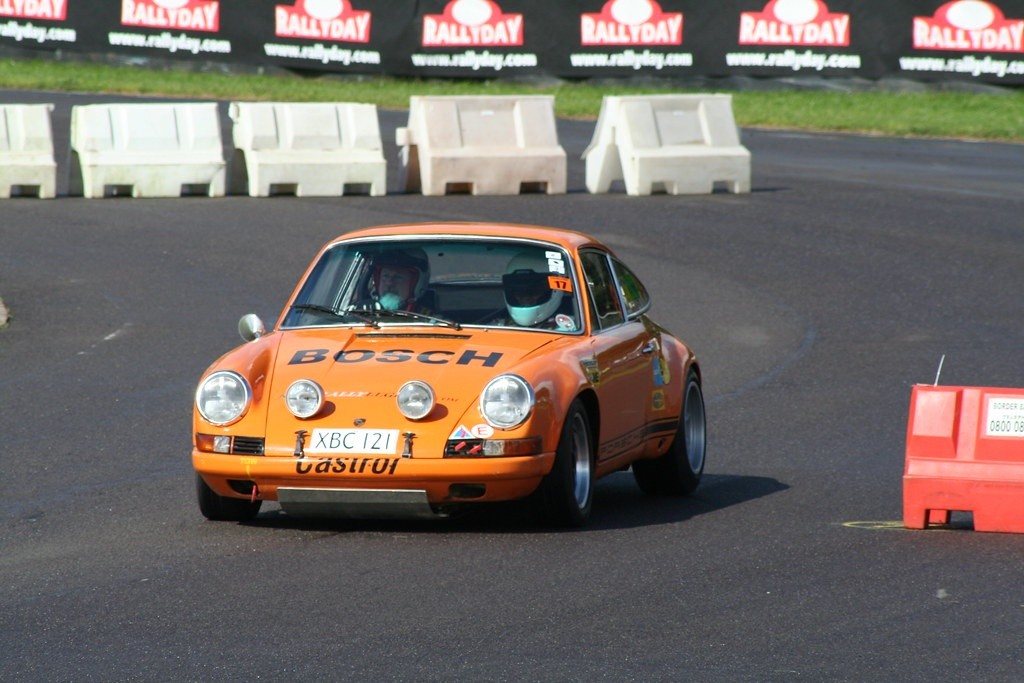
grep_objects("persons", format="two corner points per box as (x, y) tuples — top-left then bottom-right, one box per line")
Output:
(338, 246), (446, 320)
(488, 244), (579, 330)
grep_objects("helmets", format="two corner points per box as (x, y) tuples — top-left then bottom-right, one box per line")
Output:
(367, 247), (432, 304)
(500, 251), (565, 327)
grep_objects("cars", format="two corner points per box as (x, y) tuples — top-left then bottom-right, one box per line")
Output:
(187, 219), (709, 527)
